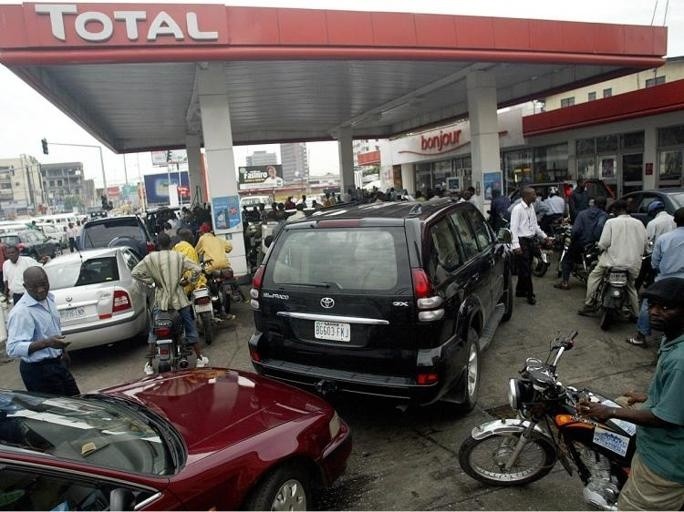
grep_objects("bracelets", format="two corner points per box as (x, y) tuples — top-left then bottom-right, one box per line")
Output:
(610, 406), (618, 418)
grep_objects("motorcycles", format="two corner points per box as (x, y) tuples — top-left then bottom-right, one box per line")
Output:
(458, 329), (638, 511)
(146, 255), (247, 376)
(493, 206), (683, 345)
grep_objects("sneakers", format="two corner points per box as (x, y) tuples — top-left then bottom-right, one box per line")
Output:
(554, 282), (568, 289)
(196, 355), (209, 367)
(143, 362), (154, 375)
(624, 336), (646, 346)
(516, 293), (535, 305)
(578, 305), (593, 313)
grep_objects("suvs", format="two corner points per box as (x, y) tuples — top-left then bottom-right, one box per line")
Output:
(249, 199), (518, 416)
(79, 215), (156, 255)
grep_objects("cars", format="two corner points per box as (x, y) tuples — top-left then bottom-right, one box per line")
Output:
(1, 366), (352, 510)
(0, 209), (182, 272)
(43, 246), (156, 352)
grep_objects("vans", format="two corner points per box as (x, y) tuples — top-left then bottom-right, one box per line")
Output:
(240, 196), (272, 211)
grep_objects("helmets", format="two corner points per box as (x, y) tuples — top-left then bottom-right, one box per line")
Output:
(549, 187), (558, 196)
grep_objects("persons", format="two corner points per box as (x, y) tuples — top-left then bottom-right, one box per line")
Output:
(30, 221), (38, 230)
(243, 191), (340, 228)
(1, 243), (44, 305)
(66, 220), (83, 252)
(5, 266), (81, 401)
(486, 178), (684, 348)
(264, 166), (284, 186)
(150, 202), (246, 319)
(341, 184), (481, 212)
(130, 231), (209, 375)
(573, 274), (684, 511)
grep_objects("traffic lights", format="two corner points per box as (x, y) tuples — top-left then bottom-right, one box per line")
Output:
(101, 195), (108, 208)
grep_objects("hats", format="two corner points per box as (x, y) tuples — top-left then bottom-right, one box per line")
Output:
(200, 225), (209, 232)
(639, 276), (683, 302)
